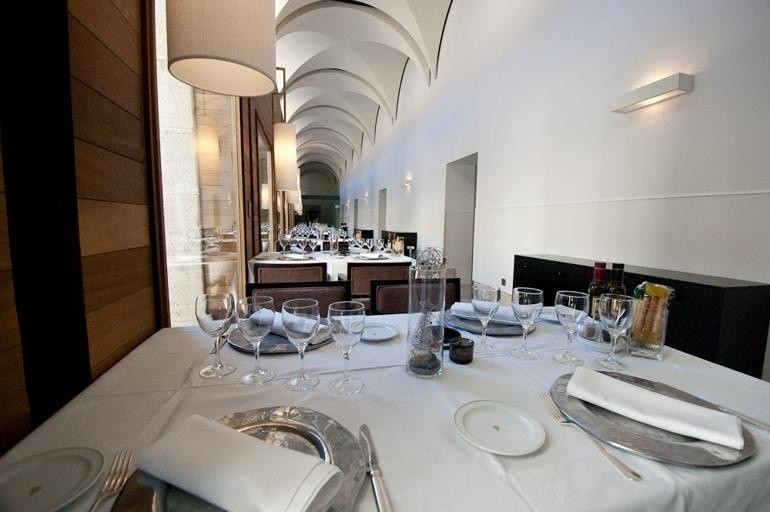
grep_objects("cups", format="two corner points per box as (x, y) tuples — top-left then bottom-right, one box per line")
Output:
(406, 267), (446, 378)
(447, 337), (474, 363)
(397, 237), (404, 256)
(627, 305), (669, 362)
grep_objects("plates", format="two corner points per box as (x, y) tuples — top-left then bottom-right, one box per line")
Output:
(0, 445), (106, 512)
(576, 332), (626, 355)
(428, 325), (461, 347)
(440, 309), (536, 337)
(112, 403), (368, 512)
(451, 398), (548, 457)
(227, 314), (332, 354)
(549, 370), (758, 468)
(360, 321), (400, 343)
(539, 308), (559, 322)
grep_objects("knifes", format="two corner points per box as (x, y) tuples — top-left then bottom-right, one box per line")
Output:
(358, 424), (392, 511)
(209, 324), (234, 354)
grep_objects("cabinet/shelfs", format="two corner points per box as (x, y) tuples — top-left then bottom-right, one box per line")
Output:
(511, 253), (769, 380)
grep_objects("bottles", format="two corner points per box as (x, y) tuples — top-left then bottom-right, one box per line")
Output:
(607, 262), (627, 295)
(387, 232), (392, 250)
(587, 259), (606, 322)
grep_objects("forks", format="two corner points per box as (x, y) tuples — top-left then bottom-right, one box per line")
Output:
(89, 445), (134, 511)
(538, 391), (641, 481)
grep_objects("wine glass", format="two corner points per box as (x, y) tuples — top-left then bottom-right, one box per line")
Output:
(281, 299), (320, 392)
(327, 301), (366, 395)
(472, 285), (501, 354)
(552, 291), (590, 366)
(196, 293), (235, 378)
(236, 294), (276, 385)
(277, 222), (385, 260)
(594, 293), (635, 371)
(510, 287), (544, 360)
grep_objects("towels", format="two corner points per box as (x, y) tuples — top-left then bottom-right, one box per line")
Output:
(565, 366), (747, 454)
(448, 300), (524, 326)
(247, 308), (334, 346)
(135, 413), (344, 512)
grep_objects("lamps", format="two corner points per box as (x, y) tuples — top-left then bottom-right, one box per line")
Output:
(273, 65), (303, 216)
(402, 179), (413, 187)
(166, 1), (278, 100)
(611, 71), (695, 116)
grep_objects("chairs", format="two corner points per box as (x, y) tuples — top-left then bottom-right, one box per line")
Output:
(244, 224), (463, 316)
